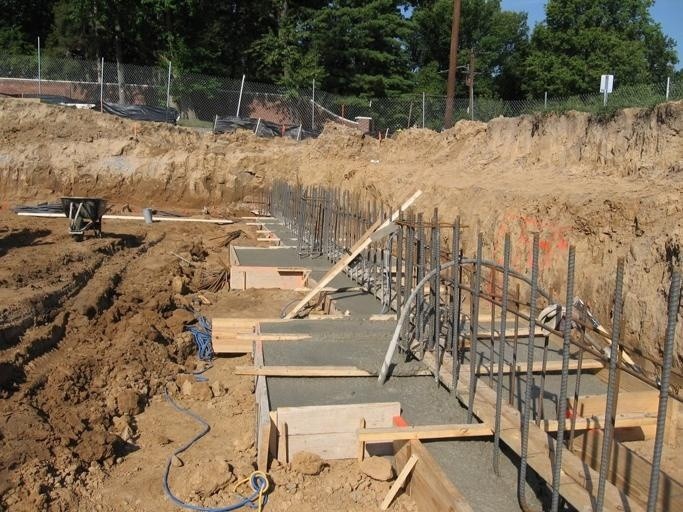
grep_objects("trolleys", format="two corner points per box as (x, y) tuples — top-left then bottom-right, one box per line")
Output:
(60, 196), (115, 239)
